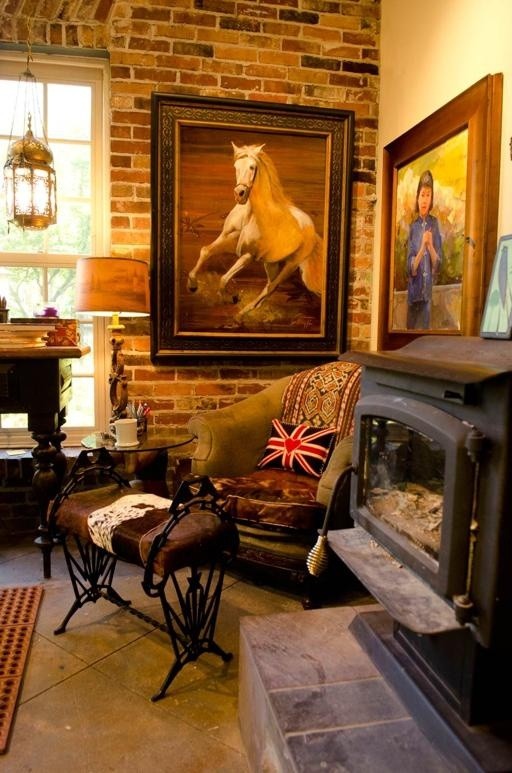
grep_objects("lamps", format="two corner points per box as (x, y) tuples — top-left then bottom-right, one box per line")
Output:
(71, 256), (151, 442)
(3, 53), (57, 231)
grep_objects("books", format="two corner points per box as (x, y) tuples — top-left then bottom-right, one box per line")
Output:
(0, 314), (62, 356)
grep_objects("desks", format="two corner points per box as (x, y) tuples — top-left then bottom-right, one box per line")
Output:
(0, 346), (91, 578)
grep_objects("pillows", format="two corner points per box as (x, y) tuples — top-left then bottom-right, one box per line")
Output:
(256, 419), (337, 479)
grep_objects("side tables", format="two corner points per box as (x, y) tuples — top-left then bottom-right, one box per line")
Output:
(82, 431), (195, 479)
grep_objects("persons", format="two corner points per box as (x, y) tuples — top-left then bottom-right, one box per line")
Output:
(405, 170), (444, 329)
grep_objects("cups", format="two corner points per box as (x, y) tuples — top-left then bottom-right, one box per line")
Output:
(109, 418), (140, 447)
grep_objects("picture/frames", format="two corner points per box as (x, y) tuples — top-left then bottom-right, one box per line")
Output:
(150, 92), (354, 366)
(480, 234), (512, 340)
(377, 73), (503, 351)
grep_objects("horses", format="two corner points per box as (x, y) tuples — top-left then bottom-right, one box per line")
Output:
(185, 140), (324, 327)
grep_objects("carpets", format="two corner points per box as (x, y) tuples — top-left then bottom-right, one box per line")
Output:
(0, 584), (44, 755)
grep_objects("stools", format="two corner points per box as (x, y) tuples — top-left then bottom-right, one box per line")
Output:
(47, 447), (240, 703)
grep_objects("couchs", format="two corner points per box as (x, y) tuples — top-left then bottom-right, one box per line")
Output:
(177, 363), (362, 610)
